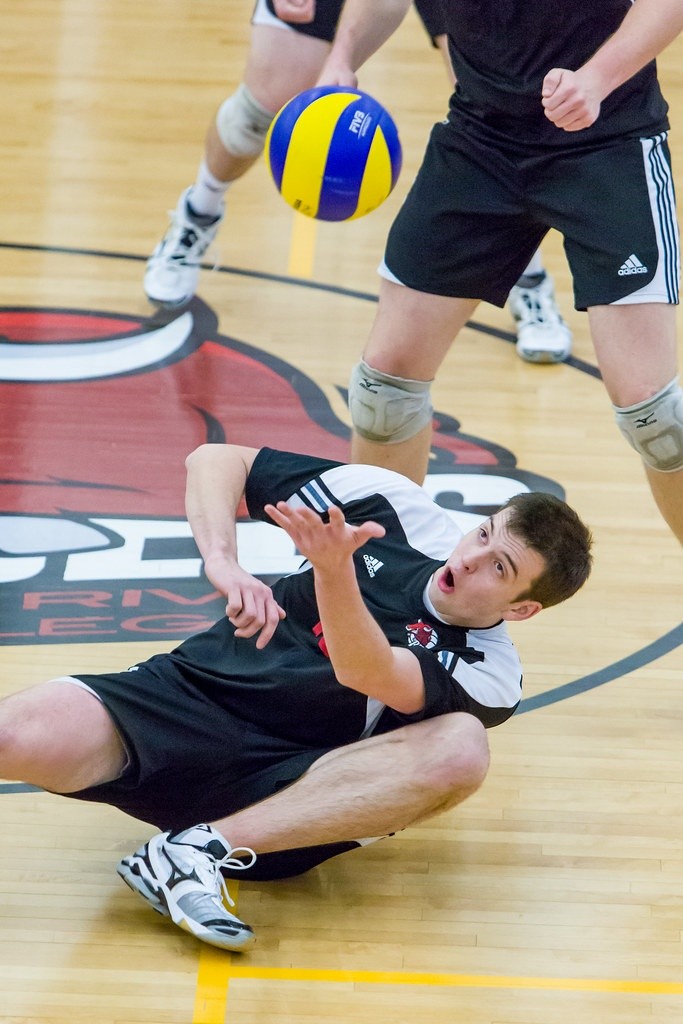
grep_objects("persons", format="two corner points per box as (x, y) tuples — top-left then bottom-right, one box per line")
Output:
(313, 1), (683, 543)
(0, 443), (594, 953)
(143, 1), (573, 364)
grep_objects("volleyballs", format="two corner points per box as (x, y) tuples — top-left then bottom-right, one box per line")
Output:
(267, 87), (400, 219)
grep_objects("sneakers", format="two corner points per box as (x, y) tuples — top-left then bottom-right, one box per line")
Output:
(508, 270), (570, 363)
(142, 186), (224, 311)
(116, 828), (256, 952)
